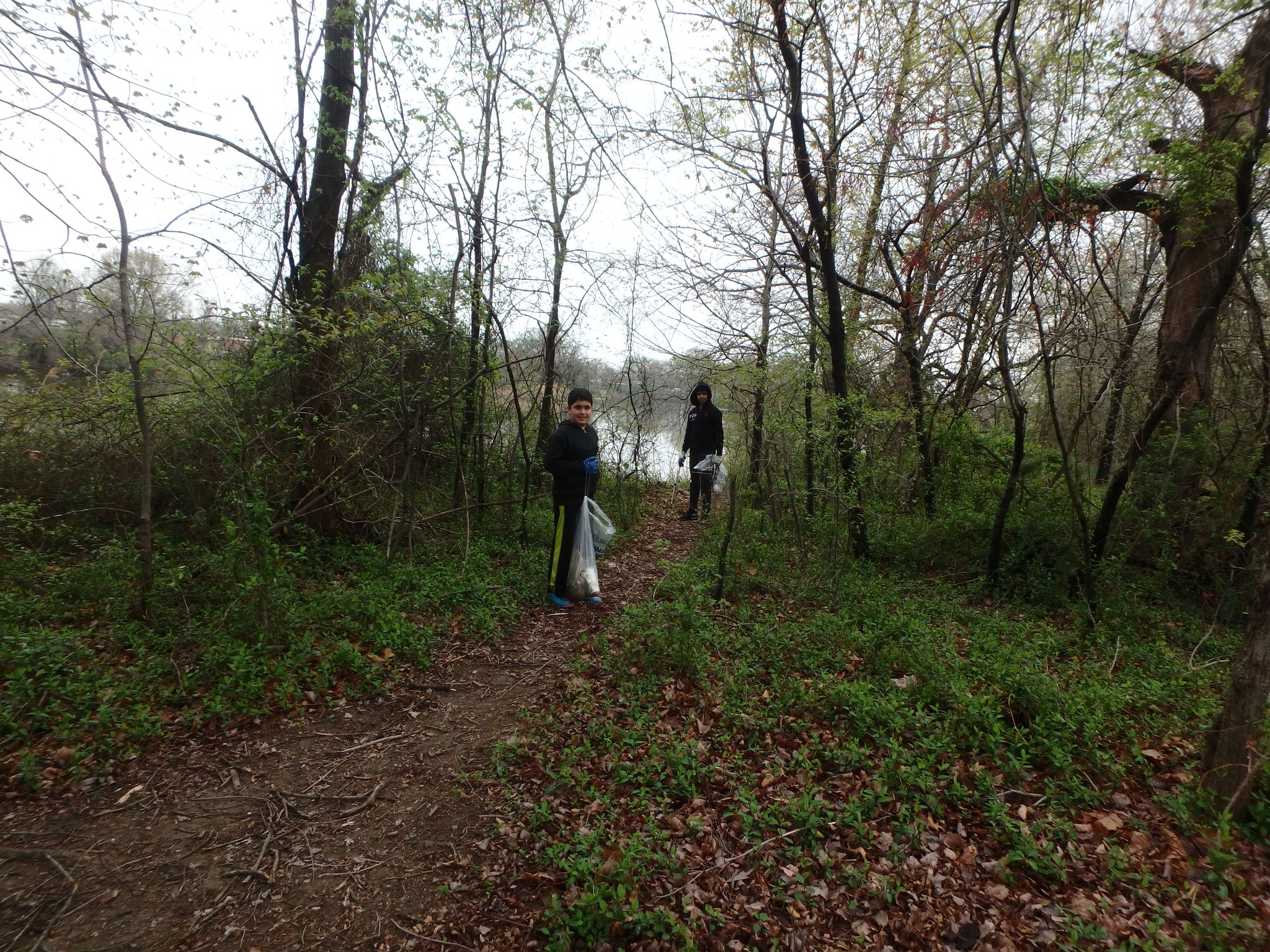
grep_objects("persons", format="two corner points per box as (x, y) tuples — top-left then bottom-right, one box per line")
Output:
(544, 388), (601, 608)
(677, 381), (724, 522)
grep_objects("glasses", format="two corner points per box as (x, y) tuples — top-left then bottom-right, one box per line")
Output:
(697, 392), (707, 396)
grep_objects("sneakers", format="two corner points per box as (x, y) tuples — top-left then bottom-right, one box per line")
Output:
(677, 511), (698, 522)
(700, 515), (709, 526)
(549, 593), (574, 608)
(583, 596), (601, 602)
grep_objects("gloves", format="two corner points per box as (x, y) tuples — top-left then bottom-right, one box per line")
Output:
(678, 451), (686, 467)
(584, 456), (599, 474)
(714, 456), (722, 467)
